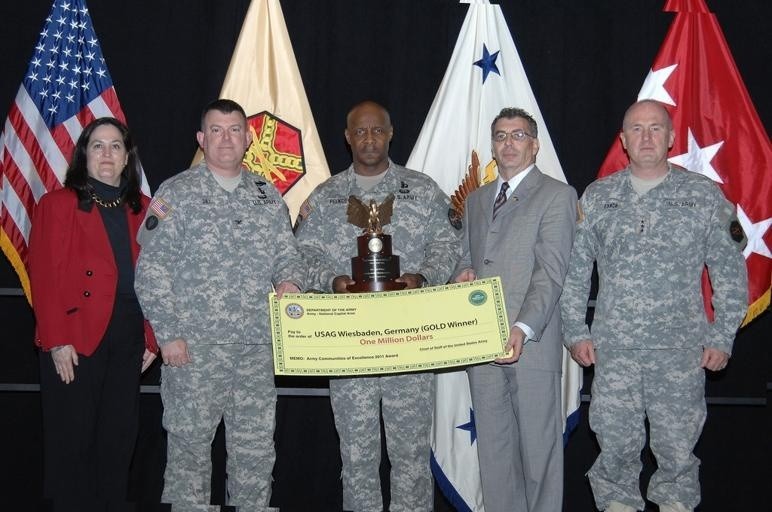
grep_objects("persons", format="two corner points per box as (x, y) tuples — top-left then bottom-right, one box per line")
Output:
(29, 116), (160, 512)
(134, 100), (303, 511)
(560, 99), (749, 512)
(453, 108), (578, 509)
(294, 101), (462, 512)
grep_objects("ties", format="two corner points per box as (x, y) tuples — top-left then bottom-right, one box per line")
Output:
(493, 183), (509, 214)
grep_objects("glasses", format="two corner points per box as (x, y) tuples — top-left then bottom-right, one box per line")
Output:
(493, 132), (536, 142)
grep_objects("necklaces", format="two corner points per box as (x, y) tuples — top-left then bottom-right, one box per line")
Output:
(91, 194), (123, 209)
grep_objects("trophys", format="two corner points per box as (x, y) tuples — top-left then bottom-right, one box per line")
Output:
(345, 192), (407, 294)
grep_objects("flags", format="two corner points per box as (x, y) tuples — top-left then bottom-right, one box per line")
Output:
(597, 1), (772, 329)
(0, 1), (152, 307)
(191, 1), (332, 228)
(404, 0), (582, 512)
